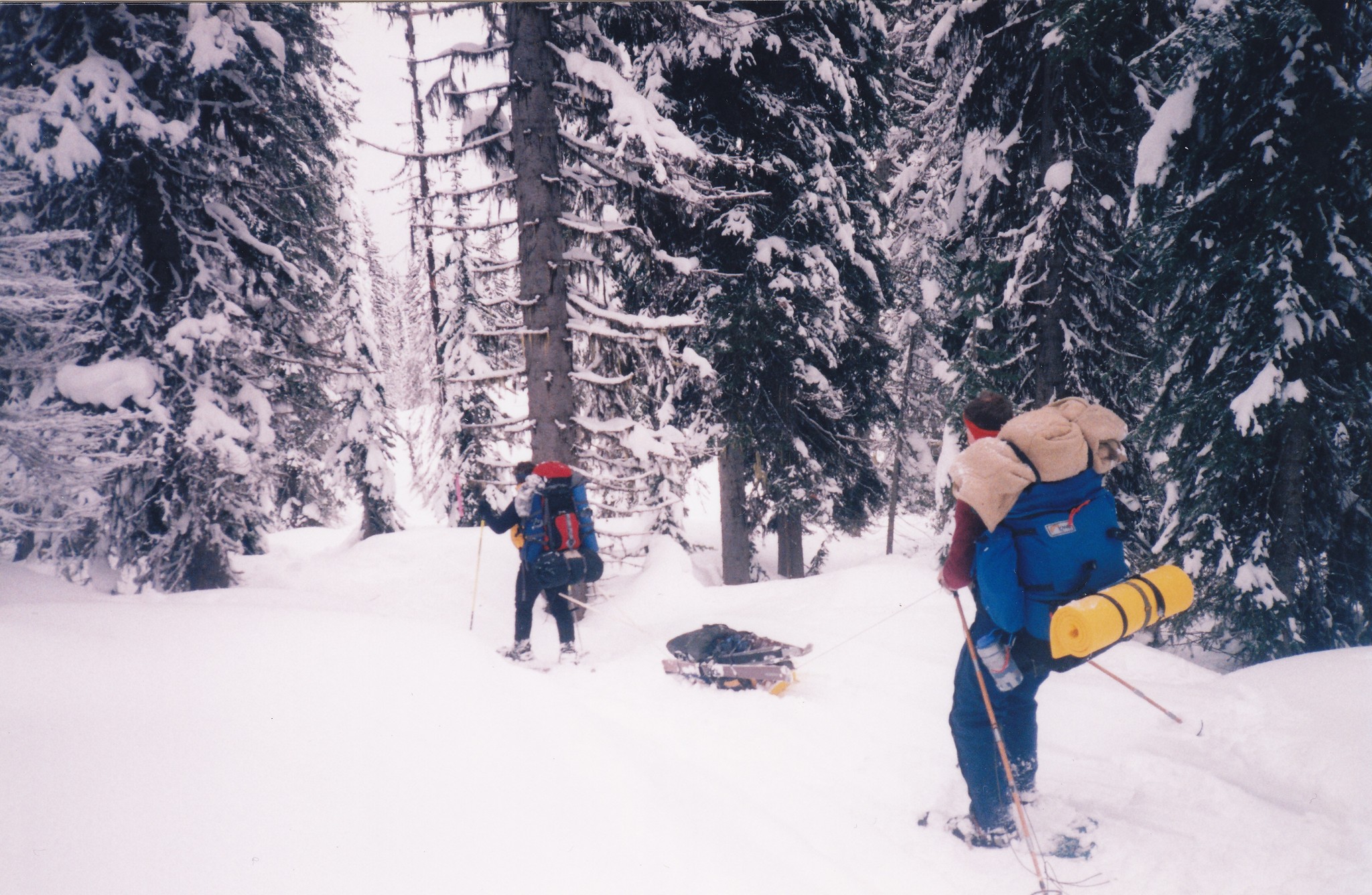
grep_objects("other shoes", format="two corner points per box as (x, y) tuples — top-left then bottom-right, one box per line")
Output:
(503, 641), (534, 661)
(948, 815), (1021, 849)
(1005, 778), (1040, 803)
(559, 642), (576, 653)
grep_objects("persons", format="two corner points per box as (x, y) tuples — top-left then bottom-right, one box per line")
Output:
(476, 462), (576, 667)
(916, 392), (1129, 848)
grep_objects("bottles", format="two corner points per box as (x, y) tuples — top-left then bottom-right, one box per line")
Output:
(976, 629), (1024, 692)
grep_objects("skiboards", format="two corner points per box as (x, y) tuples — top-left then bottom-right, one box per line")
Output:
(495, 641), (594, 675)
(905, 806), (1102, 860)
(659, 659), (793, 684)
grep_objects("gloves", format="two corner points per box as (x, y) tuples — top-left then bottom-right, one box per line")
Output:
(478, 498), (490, 512)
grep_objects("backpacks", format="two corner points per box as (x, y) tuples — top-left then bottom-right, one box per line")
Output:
(517, 487), (605, 590)
(973, 448), (1138, 672)
